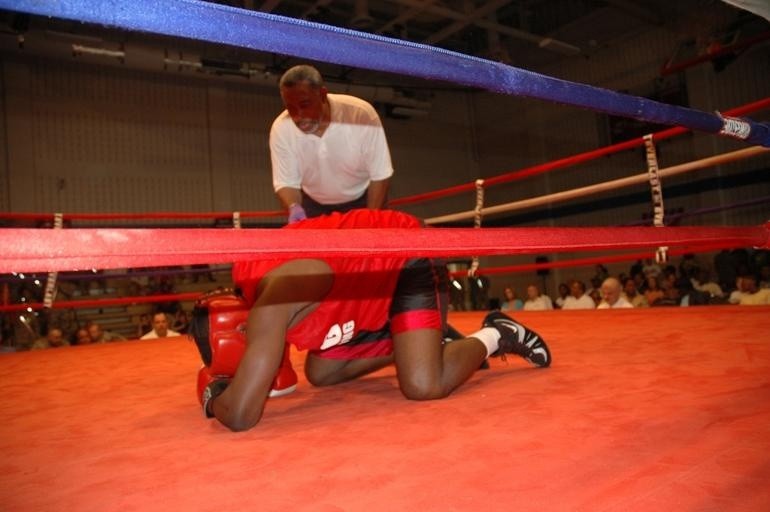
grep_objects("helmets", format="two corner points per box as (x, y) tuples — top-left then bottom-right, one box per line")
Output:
(190, 295), (252, 379)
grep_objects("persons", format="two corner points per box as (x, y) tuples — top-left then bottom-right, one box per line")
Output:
(266, 63), (396, 229)
(445, 257), (479, 311)
(555, 254), (728, 309)
(501, 285), (552, 311)
(0, 261), (221, 355)
(713, 250), (770, 305)
(187, 208), (554, 432)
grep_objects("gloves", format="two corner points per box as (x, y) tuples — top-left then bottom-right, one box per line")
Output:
(268, 366), (299, 397)
(196, 366), (228, 418)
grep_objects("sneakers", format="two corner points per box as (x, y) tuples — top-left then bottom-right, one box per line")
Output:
(441, 323), (490, 369)
(481, 309), (552, 366)
